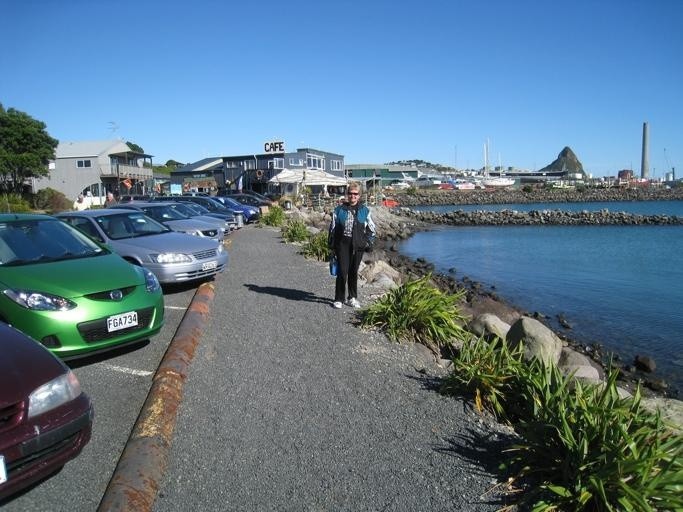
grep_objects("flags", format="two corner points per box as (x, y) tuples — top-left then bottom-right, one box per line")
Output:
(123, 179), (133, 190)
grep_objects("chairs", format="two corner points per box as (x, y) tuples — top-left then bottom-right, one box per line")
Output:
(108, 221), (127, 234)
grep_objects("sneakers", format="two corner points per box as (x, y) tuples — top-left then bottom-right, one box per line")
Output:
(333, 300), (344, 308)
(347, 297), (360, 307)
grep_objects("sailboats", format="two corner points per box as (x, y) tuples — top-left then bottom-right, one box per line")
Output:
(475, 137), (515, 187)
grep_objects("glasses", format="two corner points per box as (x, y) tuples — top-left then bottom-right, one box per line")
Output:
(348, 192), (359, 195)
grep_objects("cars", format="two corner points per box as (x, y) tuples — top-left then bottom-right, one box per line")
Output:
(0, 321), (94, 502)
(0, 213), (165, 361)
(52, 209), (227, 283)
(109, 190), (271, 244)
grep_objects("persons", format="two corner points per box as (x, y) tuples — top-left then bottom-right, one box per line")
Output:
(71, 194), (91, 212)
(327, 181), (378, 310)
(102, 192), (118, 209)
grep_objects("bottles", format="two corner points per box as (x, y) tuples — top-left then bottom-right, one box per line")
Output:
(364, 246), (373, 265)
(330, 255), (337, 276)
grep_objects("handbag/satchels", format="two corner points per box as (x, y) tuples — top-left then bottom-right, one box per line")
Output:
(329, 253), (338, 276)
